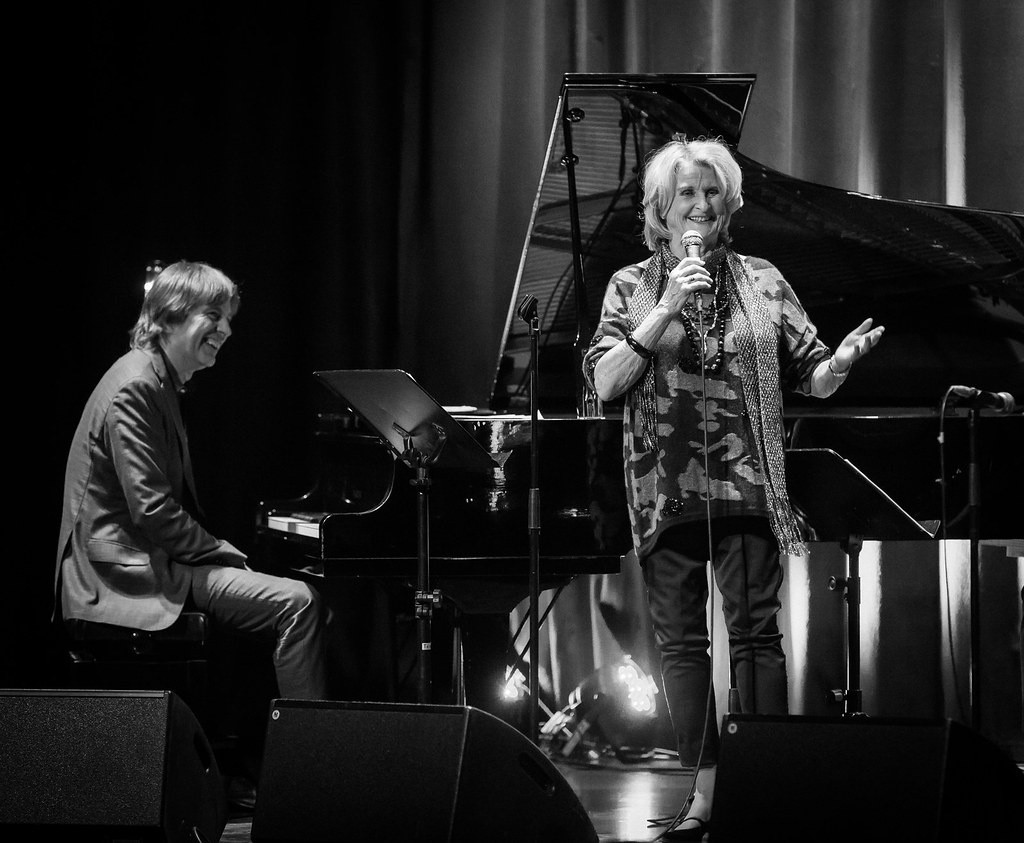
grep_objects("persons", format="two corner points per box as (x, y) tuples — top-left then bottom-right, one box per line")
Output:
(49, 260), (326, 700)
(583, 137), (884, 843)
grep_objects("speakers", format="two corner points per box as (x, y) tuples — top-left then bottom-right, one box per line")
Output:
(0, 688), (230, 843)
(250, 698), (600, 843)
(707, 713), (1024, 843)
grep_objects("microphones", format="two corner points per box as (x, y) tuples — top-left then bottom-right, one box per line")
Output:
(680, 229), (705, 311)
(952, 385), (1014, 416)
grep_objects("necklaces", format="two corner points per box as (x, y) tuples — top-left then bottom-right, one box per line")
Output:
(666, 267), (729, 373)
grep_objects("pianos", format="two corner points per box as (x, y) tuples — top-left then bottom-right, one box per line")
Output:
(244, 68), (1024, 755)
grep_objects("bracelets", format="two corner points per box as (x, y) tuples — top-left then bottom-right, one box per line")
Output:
(626, 333), (653, 360)
(829, 355), (853, 377)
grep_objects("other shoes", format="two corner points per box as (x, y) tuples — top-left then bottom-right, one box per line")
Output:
(226, 794), (258, 818)
(660, 811), (710, 843)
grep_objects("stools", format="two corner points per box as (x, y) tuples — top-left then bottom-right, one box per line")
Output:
(66, 610), (209, 716)
